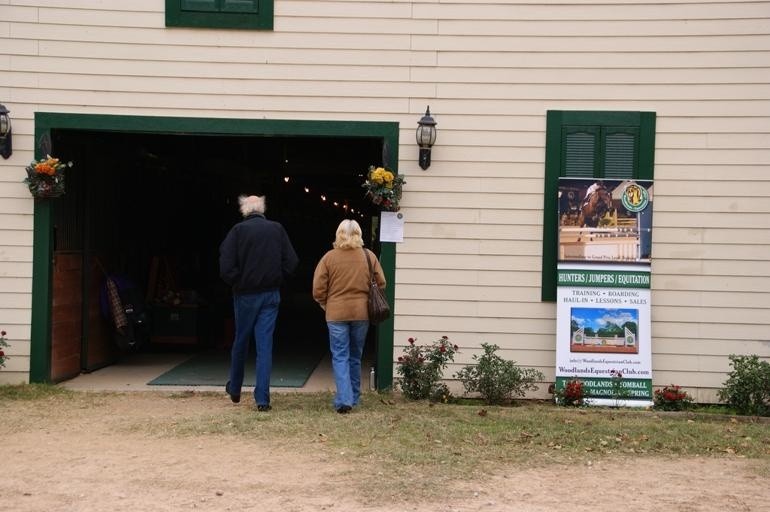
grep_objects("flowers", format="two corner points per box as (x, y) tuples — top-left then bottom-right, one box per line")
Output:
(363, 166), (406, 212)
(26, 155), (71, 198)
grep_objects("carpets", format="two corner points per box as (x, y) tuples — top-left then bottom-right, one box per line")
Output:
(147, 340), (330, 387)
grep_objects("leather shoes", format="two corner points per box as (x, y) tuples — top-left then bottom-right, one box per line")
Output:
(337, 404), (352, 413)
(226, 380), (240, 403)
(257, 404), (272, 412)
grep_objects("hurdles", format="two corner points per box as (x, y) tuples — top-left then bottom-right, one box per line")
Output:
(558, 225), (652, 262)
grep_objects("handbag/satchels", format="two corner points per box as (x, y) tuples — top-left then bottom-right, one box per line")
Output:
(369, 282), (392, 324)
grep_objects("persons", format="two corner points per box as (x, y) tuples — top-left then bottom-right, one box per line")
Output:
(216, 193), (302, 410)
(582, 181), (608, 209)
(312, 217), (390, 412)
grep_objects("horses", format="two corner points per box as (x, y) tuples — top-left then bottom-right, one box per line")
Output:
(576, 187), (614, 241)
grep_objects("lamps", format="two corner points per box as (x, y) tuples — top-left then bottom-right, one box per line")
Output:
(415, 106), (438, 170)
(0, 104), (12, 160)
